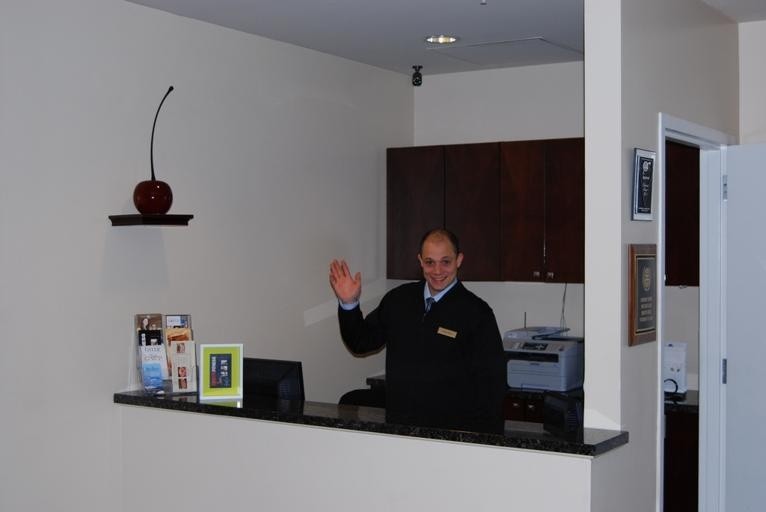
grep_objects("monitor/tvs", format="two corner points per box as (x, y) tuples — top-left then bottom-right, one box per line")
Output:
(243, 357), (305, 400)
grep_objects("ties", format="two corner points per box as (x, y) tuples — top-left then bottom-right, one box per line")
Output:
(424, 296), (435, 313)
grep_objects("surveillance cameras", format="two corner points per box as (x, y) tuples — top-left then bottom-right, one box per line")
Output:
(412, 66), (423, 86)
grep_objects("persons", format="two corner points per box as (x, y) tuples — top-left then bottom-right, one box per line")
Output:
(327, 227), (509, 420)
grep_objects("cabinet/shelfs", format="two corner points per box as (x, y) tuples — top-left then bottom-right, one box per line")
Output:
(386, 135), (699, 282)
(367, 375), (699, 512)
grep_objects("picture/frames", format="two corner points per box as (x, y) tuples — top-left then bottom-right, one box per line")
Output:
(201, 344), (246, 404)
(628, 243), (657, 347)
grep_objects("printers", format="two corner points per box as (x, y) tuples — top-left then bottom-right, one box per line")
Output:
(502, 327), (584, 392)
